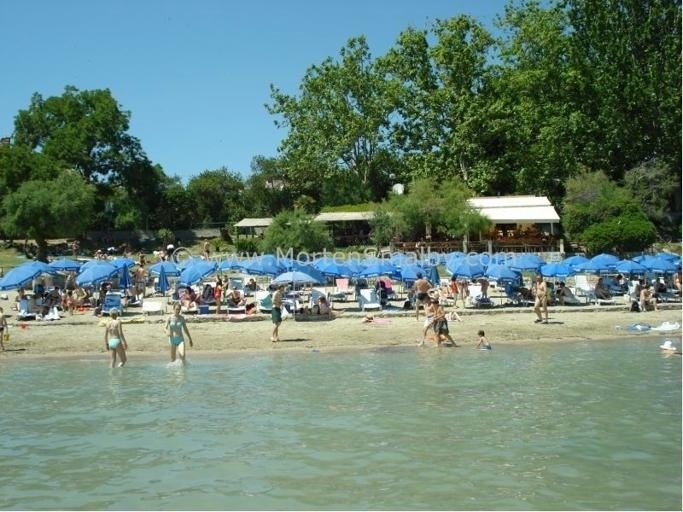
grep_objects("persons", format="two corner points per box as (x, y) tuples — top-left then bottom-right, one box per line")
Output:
(417, 295), (436, 346)
(0, 307), (9, 348)
(475, 330), (491, 350)
(104, 307), (128, 368)
(163, 302), (193, 362)
(659, 340), (682, 358)
(270, 285), (284, 342)
(432, 300), (455, 346)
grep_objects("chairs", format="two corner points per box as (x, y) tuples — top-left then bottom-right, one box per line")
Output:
(1, 263), (682, 318)
